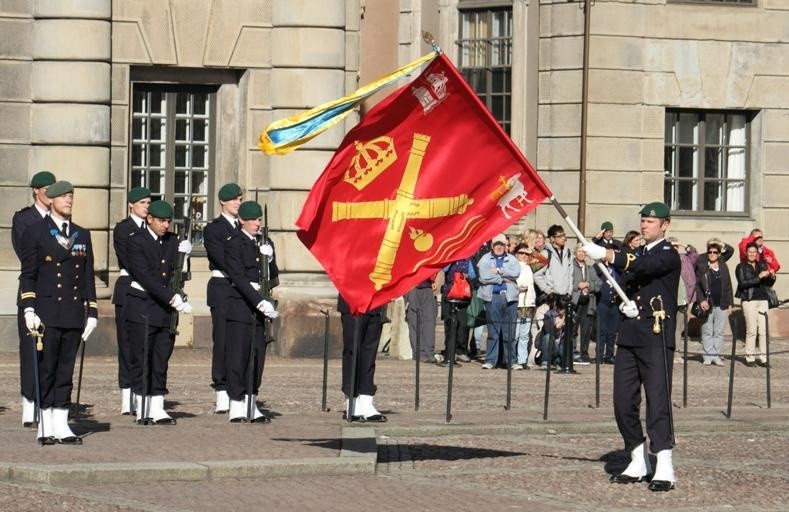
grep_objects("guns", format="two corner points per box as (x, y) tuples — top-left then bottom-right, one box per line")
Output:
(169, 196), (194, 334)
(260, 203), (277, 342)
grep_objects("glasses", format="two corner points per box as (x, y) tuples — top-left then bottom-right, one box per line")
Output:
(551, 233), (566, 238)
(707, 249), (720, 254)
(516, 249), (531, 254)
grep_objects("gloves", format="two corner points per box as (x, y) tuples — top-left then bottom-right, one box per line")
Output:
(619, 299), (641, 318)
(178, 241), (193, 254)
(22, 310), (43, 332)
(170, 293), (193, 314)
(257, 300), (279, 318)
(82, 316), (98, 341)
(260, 244), (275, 257)
(584, 242), (608, 261)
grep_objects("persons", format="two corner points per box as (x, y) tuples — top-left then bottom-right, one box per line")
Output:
(125, 201), (192, 426)
(21, 181), (99, 445)
(11, 171), (56, 427)
(337, 292), (389, 422)
(203, 184), (244, 413)
(583, 203), (681, 493)
(113, 187), (151, 416)
(223, 201), (280, 422)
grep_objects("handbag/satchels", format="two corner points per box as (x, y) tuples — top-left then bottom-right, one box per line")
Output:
(446, 272), (472, 300)
(765, 287), (779, 309)
(691, 300), (711, 324)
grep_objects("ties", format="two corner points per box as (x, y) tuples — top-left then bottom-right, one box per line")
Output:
(140, 222), (146, 232)
(234, 221), (239, 230)
(61, 223), (70, 238)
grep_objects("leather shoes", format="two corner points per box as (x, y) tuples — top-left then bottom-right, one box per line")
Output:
(747, 359), (772, 367)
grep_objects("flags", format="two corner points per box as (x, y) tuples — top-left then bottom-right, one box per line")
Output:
(295, 50), (551, 319)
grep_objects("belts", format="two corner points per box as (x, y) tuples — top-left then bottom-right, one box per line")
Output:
(211, 269), (230, 278)
(119, 268), (130, 277)
(494, 290), (507, 296)
(130, 280), (149, 293)
(232, 280), (261, 291)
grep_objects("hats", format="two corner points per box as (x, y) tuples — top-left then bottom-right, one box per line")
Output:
(238, 202), (263, 220)
(30, 171), (56, 187)
(600, 221), (614, 229)
(45, 180), (75, 199)
(148, 200), (173, 218)
(218, 183), (243, 201)
(638, 201), (670, 218)
(492, 232), (507, 245)
(127, 187), (152, 202)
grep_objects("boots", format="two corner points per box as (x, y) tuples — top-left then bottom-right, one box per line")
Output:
(245, 394), (272, 424)
(646, 450), (676, 491)
(609, 440), (654, 484)
(345, 393), (365, 422)
(229, 398), (248, 423)
(214, 389), (230, 413)
(22, 396), (84, 447)
(119, 388), (176, 426)
(359, 394), (388, 422)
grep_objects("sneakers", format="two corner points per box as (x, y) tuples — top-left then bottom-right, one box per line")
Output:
(701, 356), (726, 368)
(434, 349), (616, 370)
